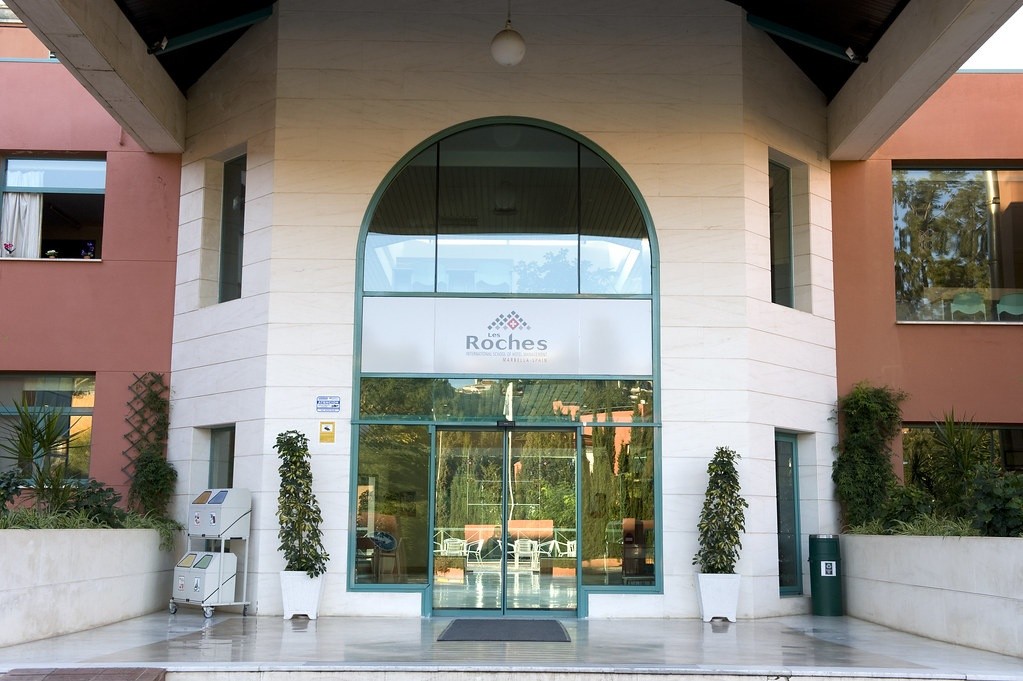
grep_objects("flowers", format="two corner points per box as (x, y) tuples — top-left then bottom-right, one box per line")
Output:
(81, 242), (95, 256)
(46, 250), (58, 256)
(3, 243), (16, 253)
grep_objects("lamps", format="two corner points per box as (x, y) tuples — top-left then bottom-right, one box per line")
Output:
(491, 1), (526, 68)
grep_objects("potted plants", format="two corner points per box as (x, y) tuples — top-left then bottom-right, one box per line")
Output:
(273, 429), (330, 619)
(692, 444), (749, 623)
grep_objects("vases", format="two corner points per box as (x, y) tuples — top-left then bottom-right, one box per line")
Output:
(50, 255), (54, 259)
(6, 251), (14, 257)
(85, 255), (90, 259)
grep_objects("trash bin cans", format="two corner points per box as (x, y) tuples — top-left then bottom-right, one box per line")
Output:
(808, 533), (844, 616)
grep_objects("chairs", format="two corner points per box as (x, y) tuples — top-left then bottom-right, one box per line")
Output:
(950, 292), (987, 322)
(996, 293), (1023, 322)
(434, 536), (576, 580)
